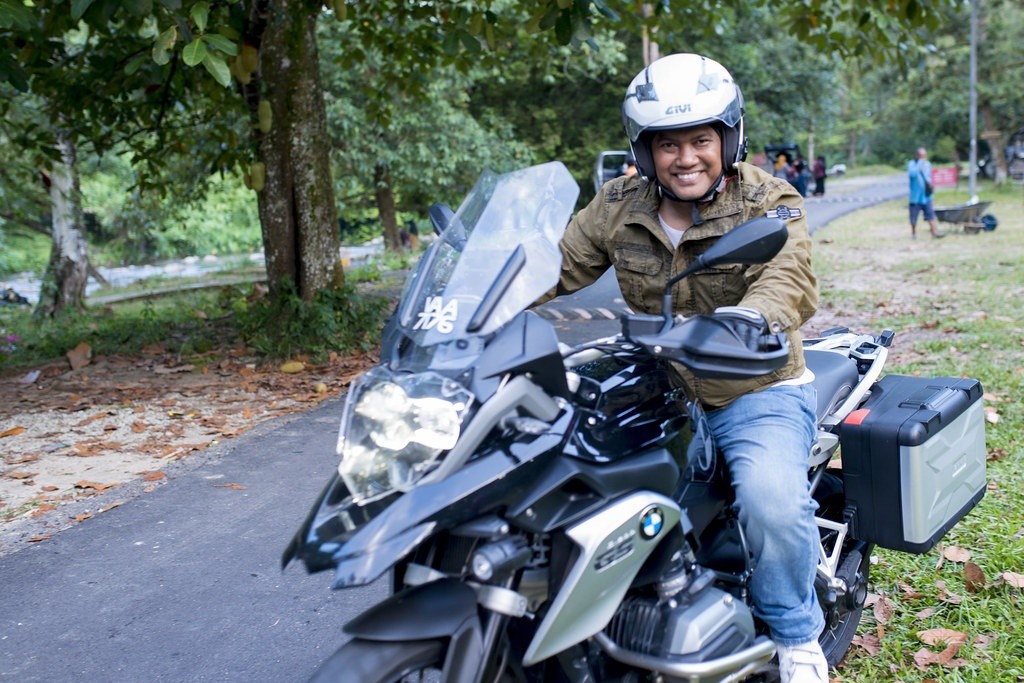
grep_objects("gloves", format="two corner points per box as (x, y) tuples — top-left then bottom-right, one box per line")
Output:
(710, 304), (770, 352)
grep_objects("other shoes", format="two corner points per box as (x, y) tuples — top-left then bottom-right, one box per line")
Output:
(912, 234), (917, 239)
(933, 232), (946, 238)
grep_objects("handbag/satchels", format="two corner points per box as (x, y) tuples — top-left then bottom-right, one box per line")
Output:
(925, 181), (934, 196)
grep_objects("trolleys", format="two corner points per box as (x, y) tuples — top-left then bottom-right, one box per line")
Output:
(928, 199), (998, 237)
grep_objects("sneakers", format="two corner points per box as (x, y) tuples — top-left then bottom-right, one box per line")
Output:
(777, 638), (829, 683)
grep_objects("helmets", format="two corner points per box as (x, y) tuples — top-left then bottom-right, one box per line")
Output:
(621, 53), (748, 185)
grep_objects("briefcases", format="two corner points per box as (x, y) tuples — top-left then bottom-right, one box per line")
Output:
(838, 374), (988, 554)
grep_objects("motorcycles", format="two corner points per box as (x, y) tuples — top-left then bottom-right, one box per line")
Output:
(280, 160), (986, 682)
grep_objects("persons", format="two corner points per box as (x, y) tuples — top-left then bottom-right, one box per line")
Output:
(907, 147), (947, 238)
(749, 150), (827, 195)
(426, 50), (831, 683)
(614, 150), (638, 180)
